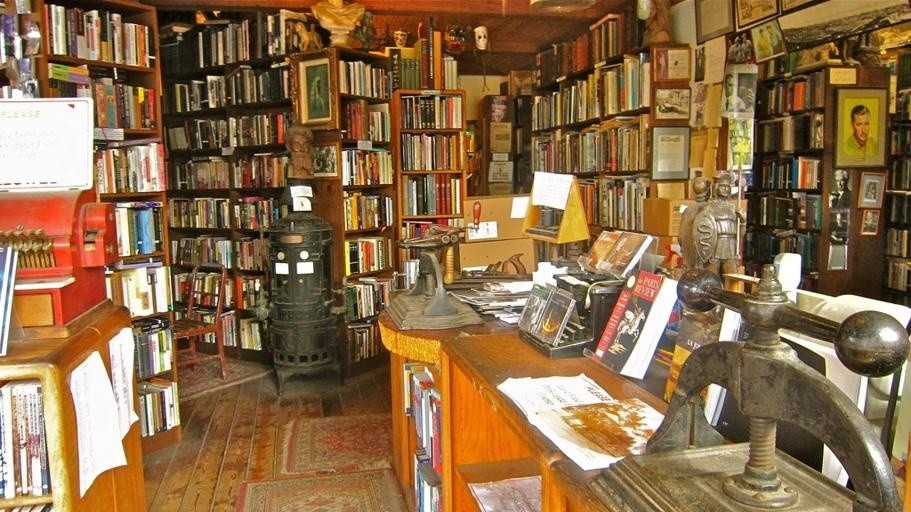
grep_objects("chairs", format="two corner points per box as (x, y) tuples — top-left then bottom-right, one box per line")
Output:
(171, 259), (229, 389)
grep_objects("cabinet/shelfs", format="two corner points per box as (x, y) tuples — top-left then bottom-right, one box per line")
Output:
(1, 1), (188, 510)
(461, 38), (907, 305)
(366, 266), (556, 510)
(292, 24), (459, 387)
(163, 46), (289, 367)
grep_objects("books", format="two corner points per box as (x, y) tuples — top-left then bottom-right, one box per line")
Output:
(1, 2), (183, 435)
(1, 243), (54, 512)
(516, 228), (741, 426)
(339, 28), (463, 363)
(169, 9), (316, 349)
(403, 362), (444, 512)
(488, 14), (654, 270)
(739, 45), (910, 307)
